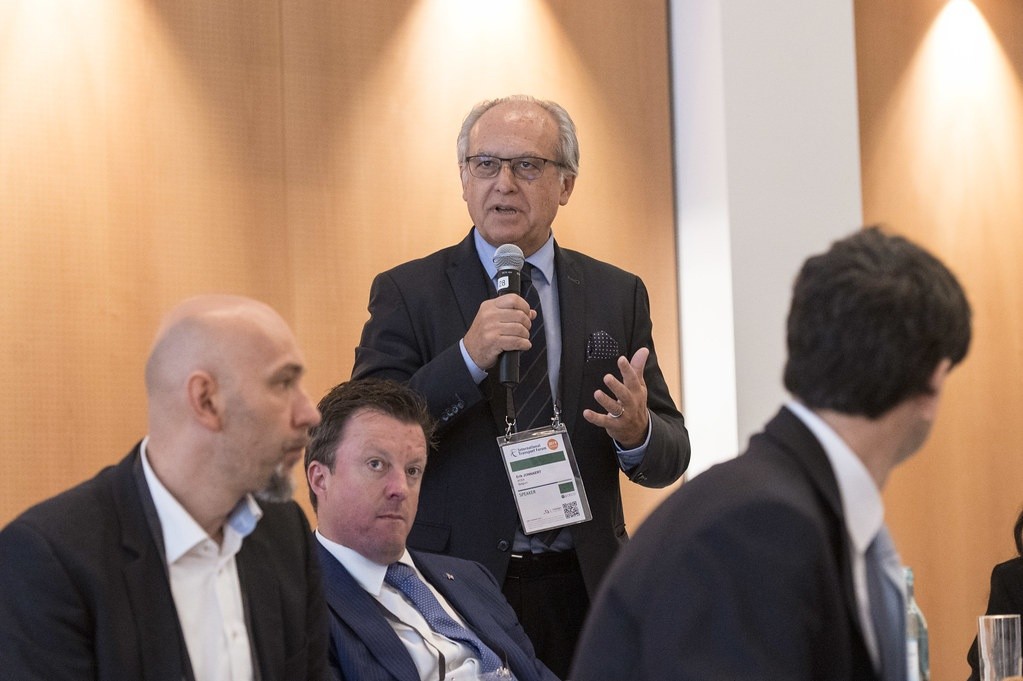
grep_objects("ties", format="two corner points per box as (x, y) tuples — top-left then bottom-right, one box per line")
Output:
(865, 532), (908, 681)
(493, 260), (564, 547)
(384, 562), (520, 681)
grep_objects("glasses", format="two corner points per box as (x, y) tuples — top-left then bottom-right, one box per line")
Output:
(466, 155), (568, 180)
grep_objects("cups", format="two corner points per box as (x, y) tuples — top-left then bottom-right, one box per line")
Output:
(978, 614), (1023, 681)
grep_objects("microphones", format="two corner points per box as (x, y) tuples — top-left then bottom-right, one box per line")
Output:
(492, 243), (526, 385)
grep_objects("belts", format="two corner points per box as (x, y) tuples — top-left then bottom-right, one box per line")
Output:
(506, 549), (579, 579)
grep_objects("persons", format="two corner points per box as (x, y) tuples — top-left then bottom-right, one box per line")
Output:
(351, 97), (692, 681)
(0, 294), (331, 681)
(305, 378), (562, 681)
(967, 510), (1023, 681)
(568, 227), (971, 681)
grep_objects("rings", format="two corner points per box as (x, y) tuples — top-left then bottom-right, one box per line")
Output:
(609, 409), (624, 418)
